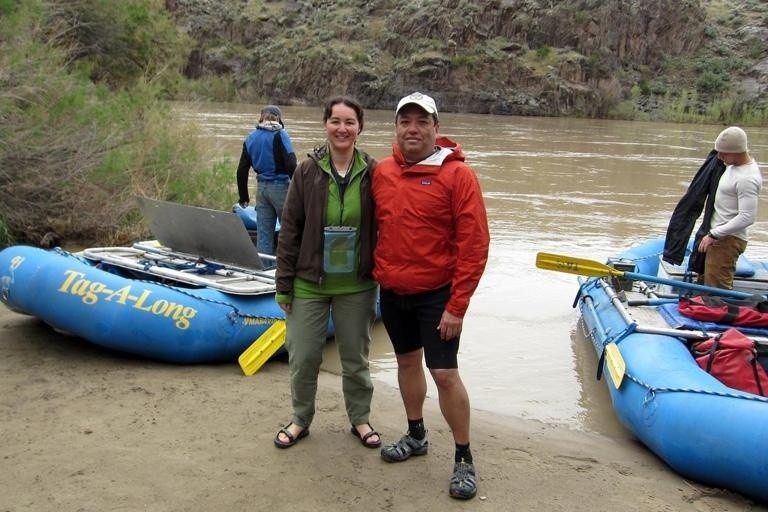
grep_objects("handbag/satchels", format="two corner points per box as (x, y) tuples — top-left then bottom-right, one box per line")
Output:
(692, 328), (768, 398)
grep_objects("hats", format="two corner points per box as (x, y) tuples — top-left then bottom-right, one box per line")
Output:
(261, 105), (285, 129)
(714, 126), (747, 153)
(395, 92), (438, 119)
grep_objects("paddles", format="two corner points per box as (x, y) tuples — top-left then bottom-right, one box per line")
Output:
(535, 251), (768, 303)
(238, 320), (287, 376)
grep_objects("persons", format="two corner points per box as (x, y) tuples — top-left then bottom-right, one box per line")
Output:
(236, 104), (299, 269)
(695, 125), (763, 290)
(272, 95), (382, 449)
(372, 91), (490, 501)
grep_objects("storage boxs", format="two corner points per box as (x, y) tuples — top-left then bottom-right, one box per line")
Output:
(656, 262), (768, 309)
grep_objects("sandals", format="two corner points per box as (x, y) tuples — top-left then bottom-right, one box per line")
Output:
(381, 432), (429, 462)
(274, 421), (310, 447)
(351, 421), (380, 447)
(449, 458), (477, 500)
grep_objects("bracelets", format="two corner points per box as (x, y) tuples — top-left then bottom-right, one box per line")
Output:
(707, 230), (719, 242)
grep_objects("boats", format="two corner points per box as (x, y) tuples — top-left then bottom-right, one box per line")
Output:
(572, 236), (767, 502)
(0, 205), (382, 367)
